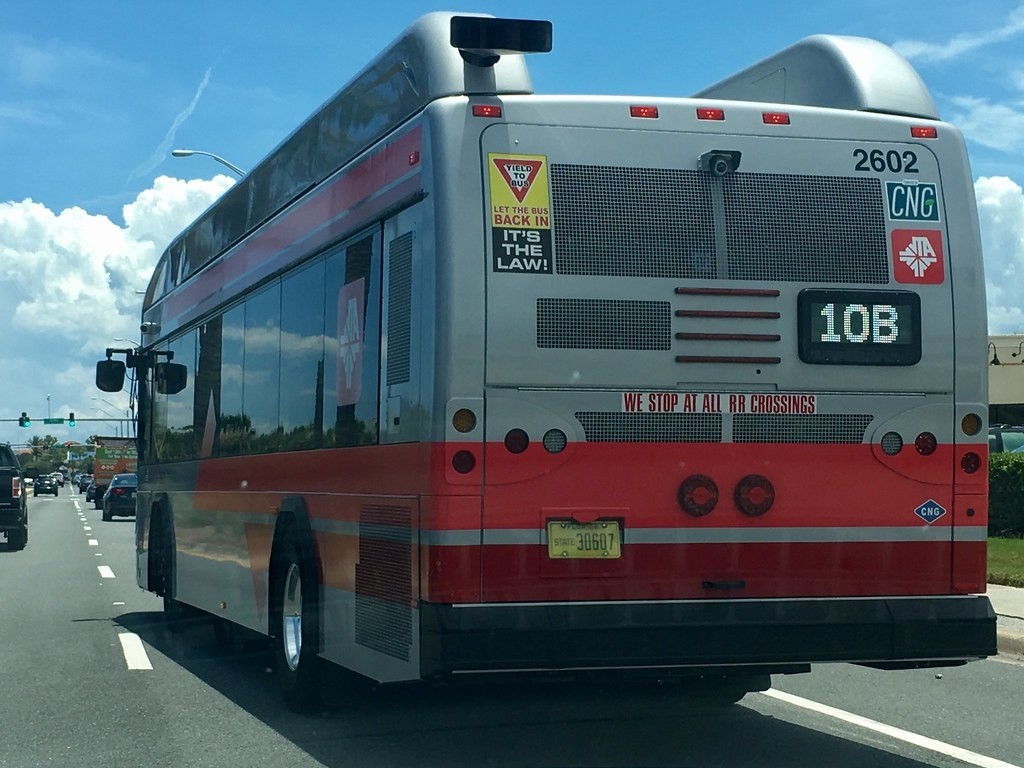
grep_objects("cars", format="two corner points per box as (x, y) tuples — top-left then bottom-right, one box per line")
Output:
(100, 472), (138, 523)
(989, 424), (1024, 456)
(71, 435), (138, 512)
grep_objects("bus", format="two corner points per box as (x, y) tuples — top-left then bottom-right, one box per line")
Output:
(92, 10), (1003, 716)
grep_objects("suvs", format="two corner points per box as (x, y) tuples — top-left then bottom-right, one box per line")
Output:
(0, 443), (40, 550)
(49, 471), (66, 487)
(33, 474), (60, 497)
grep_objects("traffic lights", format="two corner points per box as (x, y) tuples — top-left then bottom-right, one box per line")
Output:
(19, 412), (30, 427)
(70, 412), (75, 427)
